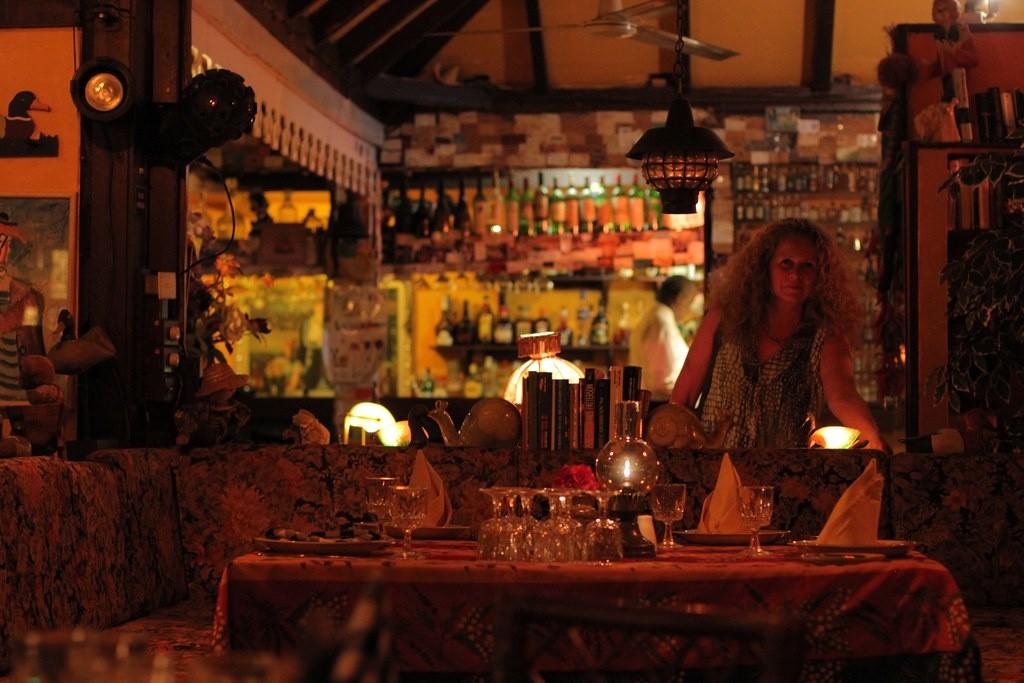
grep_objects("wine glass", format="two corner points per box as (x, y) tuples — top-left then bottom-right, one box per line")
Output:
(650, 485), (686, 550)
(386, 268), (556, 296)
(364, 477), (428, 561)
(736, 487), (775, 557)
(478, 488), (624, 561)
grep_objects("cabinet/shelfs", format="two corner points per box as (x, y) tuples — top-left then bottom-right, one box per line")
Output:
(731, 158), (909, 410)
(193, 159), (713, 278)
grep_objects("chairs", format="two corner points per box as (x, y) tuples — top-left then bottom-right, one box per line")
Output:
(490, 592), (809, 683)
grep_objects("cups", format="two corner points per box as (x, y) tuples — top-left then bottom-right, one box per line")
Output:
(26, 636), (294, 683)
(638, 516), (657, 553)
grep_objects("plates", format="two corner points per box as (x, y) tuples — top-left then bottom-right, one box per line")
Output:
(358, 524), (469, 540)
(254, 538), (388, 554)
(674, 530), (789, 543)
(792, 541), (918, 557)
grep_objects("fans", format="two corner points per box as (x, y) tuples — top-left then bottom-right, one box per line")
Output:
(423, 0), (738, 61)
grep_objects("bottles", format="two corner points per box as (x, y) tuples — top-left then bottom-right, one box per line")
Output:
(567, 174), (663, 234)
(190, 179), (246, 259)
(411, 354), (522, 397)
(433, 291), (533, 343)
(385, 177), (487, 240)
(277, 191), (324, 233)
(734, 163), (879, 194)
(833, 227), (882, 340)
(734, 192), (878, 225)
(488, 173), (567, 237)
(255, 102), (380, 197)
(853, 344), (883, 402)
(191, 47), (222, 81)
(537, 289), (634, 348)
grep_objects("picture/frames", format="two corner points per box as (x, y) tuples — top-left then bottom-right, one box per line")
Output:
(0, 192), (77, 407)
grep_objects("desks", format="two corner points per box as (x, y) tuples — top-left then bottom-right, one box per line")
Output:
(209, 541), (973, 672)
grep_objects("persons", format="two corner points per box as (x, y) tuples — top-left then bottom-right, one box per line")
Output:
(673, 219), (894, 453)
(628, 275), (697, 406)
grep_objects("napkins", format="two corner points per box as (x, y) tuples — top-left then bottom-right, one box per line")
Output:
(817, 458), (885, 545)
(696, 452), (745, 534)
(410, 449), (453, 528)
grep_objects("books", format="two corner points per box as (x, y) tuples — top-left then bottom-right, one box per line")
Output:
(949, 158), (1004, 228)
(521, 365), (651, 449)
(952, 69), (1024, 144)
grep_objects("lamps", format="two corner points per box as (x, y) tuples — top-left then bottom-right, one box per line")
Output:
(173, 67), (259, 167)
(70, 58), (137, 122)
(624, 0), (738, 214)
(505, 330), (585, 402)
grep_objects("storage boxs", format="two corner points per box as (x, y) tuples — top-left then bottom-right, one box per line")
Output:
(260, 224), (301, 263)
(384, 109), (883, 160)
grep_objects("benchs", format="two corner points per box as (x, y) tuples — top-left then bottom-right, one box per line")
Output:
(0, 439), (1024, 683)
